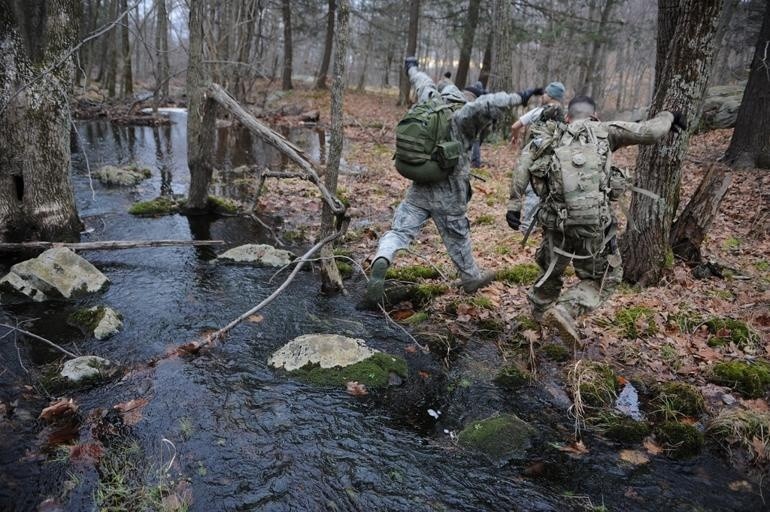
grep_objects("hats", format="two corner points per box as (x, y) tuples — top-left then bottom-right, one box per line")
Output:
(545, 81), (565, 100)
(444, 71), (451, 78)
(465, 81), (489, 97)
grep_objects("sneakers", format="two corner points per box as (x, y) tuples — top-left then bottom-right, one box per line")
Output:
(366, 257), (388, 307)
(540, 304), (583, 352)
(462, 269), (495, 294)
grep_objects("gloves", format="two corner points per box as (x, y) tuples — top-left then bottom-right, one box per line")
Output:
(670, 110), (687, 134)
(523, 87), (544, 98)
(404, 56), (419, 68)
(506, 210), (520, 230)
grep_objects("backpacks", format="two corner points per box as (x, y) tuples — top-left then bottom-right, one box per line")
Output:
(611, 164), (626, 199)
(392, 99), (463, 184)
(537, 142), (610, 239)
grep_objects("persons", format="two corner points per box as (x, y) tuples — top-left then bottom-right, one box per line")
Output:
(437, 71), (457, 92)
(462, 80), (489, 170)
(367, 57), (541, 301)
